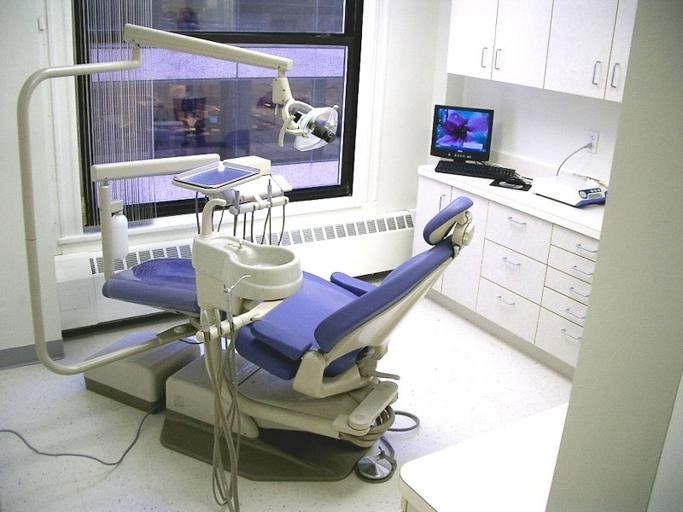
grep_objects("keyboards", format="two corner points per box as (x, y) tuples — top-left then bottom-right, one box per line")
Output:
(434, 159), (517, 180)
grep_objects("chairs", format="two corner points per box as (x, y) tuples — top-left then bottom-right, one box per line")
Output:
(101, 194), (474, 483)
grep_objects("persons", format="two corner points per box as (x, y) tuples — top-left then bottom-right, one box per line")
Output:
(182, 110), (207, 148)
(177, 8), (200, 30)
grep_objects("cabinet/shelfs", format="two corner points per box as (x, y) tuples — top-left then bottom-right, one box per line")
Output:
(411, 173), (601, 370)
(445, 1), (637, 104)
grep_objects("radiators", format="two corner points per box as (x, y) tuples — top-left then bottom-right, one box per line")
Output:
(53, 207), (414, 330)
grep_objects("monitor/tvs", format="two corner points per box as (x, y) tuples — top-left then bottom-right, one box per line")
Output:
(430, 104), (495, 163)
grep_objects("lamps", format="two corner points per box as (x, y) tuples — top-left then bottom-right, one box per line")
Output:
(16, 22), (339, 376)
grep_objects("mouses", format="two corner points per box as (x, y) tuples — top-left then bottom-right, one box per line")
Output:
(497, 177), (525, 190)
